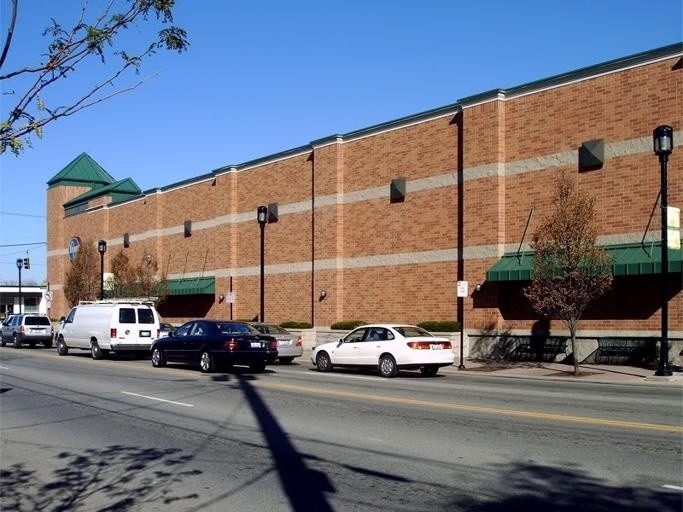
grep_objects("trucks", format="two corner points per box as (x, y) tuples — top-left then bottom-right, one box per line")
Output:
(56, 300), (160, 359)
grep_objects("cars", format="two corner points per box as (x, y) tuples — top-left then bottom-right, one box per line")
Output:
(158, 322), (176, 339)
(149, 320), (278, 373)
(309, 323), (454, 378)
(245, 322), (303, 365)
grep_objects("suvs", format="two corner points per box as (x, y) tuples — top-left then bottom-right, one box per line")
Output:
(0, 314), (64, 346)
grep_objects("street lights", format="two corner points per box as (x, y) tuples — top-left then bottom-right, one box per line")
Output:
(16, 258), (22, 313)
(256, 206), (267, 323)
(653, 124), (673, 376)
(98, 240), (106, 300)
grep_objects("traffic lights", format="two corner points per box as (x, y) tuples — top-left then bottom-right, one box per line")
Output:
(23, 257), (29, 270)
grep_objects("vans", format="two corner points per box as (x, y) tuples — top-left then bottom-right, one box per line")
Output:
(1, 312), (53, 348)
